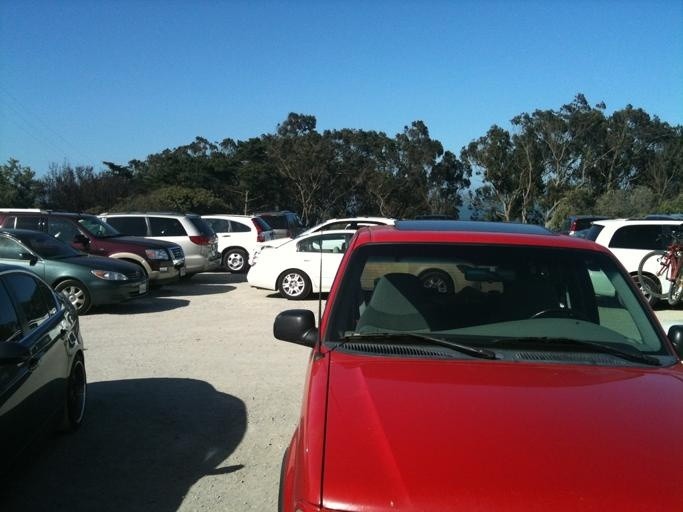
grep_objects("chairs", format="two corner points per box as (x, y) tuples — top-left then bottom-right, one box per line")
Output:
(357, 269), (564, 338)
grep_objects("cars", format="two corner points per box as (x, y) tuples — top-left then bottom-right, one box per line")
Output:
(2, 265), (89, 477)
